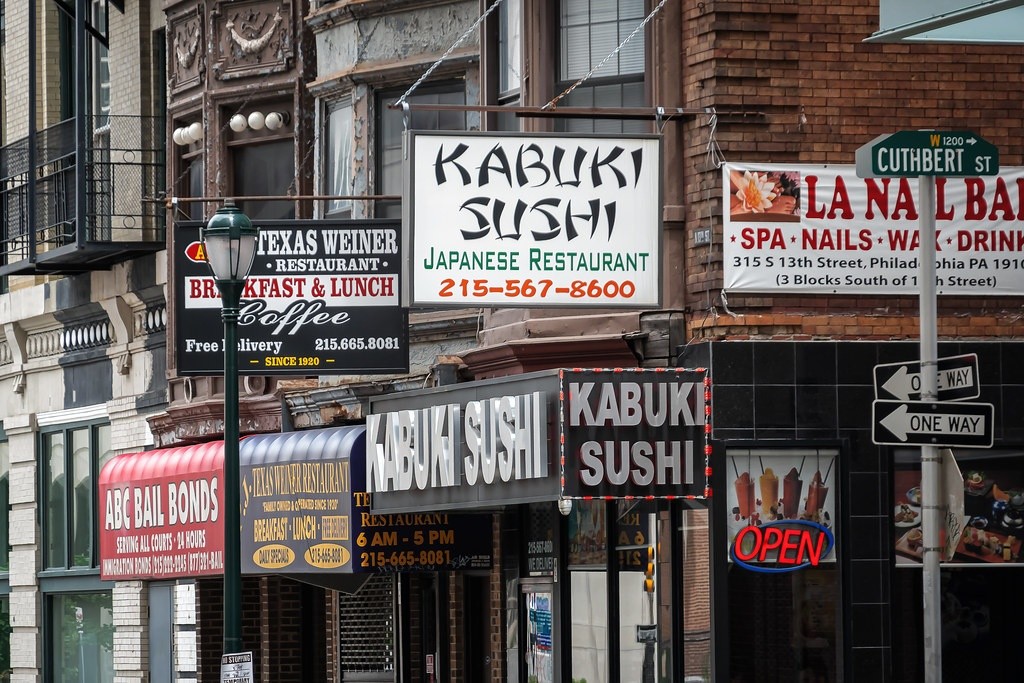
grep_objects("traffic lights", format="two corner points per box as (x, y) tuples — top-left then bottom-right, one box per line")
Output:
(642, 547), (656, 593)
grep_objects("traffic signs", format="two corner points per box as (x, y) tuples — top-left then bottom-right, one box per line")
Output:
(868, 129), (998, 178)
(873, 353), (982, 403)
(872, 400), (997, 449)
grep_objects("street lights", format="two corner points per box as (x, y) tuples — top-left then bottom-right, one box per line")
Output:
(192, 198), (258, 683)
